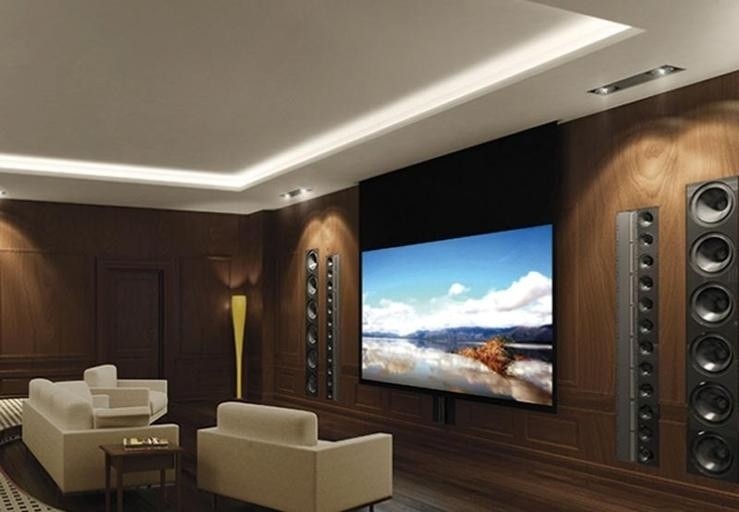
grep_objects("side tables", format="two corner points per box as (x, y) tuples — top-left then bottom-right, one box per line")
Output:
(99, 441), (183, 512)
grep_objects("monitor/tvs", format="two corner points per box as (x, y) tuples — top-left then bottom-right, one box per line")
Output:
(359, 223), (558, 415)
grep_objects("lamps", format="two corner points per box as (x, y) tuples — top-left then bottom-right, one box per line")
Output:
(230, 295), (247, 399)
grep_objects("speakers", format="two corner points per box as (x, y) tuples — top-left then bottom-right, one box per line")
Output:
(304, 247), (320, 397)
(326, 253), (341, 403)
(614, 207), (660, 467)
(684, 176), (739, 482)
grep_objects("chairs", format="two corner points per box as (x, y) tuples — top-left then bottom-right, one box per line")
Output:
(84, 364), (169, 425)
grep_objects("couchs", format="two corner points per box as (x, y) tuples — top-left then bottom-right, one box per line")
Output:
(22, 378), (180, 512)
(196, 401), (393, 510)
